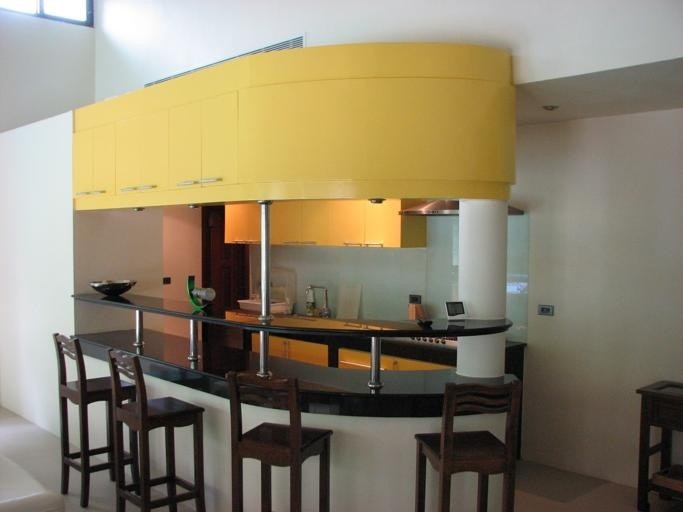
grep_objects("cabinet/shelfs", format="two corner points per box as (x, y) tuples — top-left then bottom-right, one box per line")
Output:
(74, 48), (304, 210)
(304, 46), (518, 200)
(249, 319), (332, 368)
(333, 332), (458, 372)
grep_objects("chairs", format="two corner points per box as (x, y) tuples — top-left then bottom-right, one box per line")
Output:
(226, 372), (333, 512)
(413, 379), (523, 512)
(53, 332), (142, 508)
(105, 347), (208, 512)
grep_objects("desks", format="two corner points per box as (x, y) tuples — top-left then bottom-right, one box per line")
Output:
(635, 380), (683, 512)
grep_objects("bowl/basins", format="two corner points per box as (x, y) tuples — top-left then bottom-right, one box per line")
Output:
(87, 279), (137, 297)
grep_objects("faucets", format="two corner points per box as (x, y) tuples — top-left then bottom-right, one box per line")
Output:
(308, 285), (331, 318)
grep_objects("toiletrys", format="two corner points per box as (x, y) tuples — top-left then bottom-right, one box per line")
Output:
(305, 288), (316, 317)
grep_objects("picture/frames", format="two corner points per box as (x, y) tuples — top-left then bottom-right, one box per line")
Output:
(305, 285), (329, 319)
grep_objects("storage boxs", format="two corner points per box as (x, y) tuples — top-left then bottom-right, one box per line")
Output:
(236, 297), (294, 315)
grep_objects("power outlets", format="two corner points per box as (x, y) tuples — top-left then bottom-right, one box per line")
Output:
(537, 305), (554, 316)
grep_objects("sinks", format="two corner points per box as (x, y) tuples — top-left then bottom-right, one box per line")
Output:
(289, 317), (320, 319)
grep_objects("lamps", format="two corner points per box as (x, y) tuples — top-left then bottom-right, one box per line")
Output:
(187, 272), (216, 315)
